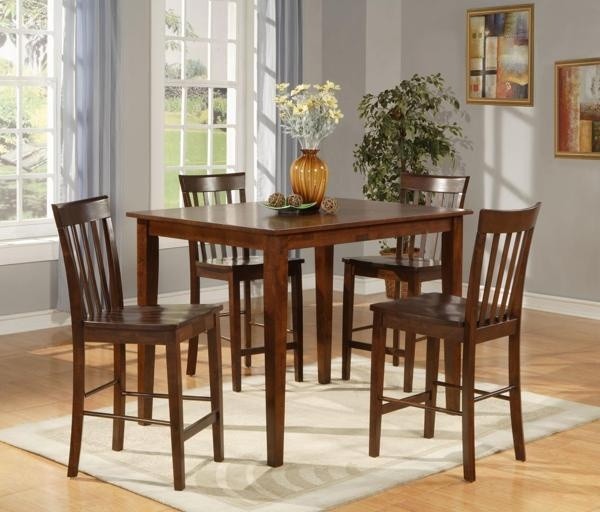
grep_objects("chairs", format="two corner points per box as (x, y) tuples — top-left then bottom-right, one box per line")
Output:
(368, 202), (541, 483)
(179, 172), (305, 392)
(342, 174), (471, 392)
(51, 195), (225, 490)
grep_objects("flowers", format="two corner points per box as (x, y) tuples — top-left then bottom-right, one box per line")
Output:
(270, 80), (343, 150)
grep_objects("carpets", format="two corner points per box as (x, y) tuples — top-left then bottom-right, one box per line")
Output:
(0, 352), (599, 511)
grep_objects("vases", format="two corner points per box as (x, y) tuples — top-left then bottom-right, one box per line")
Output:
(289, 150), (329, 213)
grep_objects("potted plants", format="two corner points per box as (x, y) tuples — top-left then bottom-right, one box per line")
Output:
(352, 72), (463, 299)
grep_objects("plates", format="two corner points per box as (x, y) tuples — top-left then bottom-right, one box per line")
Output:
(259, 201), (317, 214)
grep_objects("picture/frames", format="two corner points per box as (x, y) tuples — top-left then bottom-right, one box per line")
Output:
(465, 4), (535, 108)
(553, 58), (599, 160)
